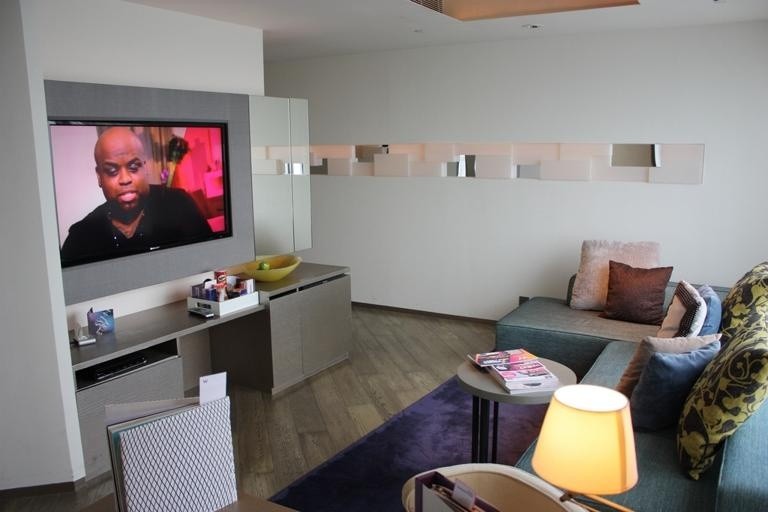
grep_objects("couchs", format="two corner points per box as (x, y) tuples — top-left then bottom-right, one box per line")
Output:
(490, 277), (768, 512)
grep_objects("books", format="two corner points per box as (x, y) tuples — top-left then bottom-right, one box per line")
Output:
(472, 347), (561, 396)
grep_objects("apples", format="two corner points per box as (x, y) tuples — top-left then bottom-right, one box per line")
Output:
(260, 262), (270, 270)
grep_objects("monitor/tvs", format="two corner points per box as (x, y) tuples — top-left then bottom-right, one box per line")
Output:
(47, 120), (234, 268)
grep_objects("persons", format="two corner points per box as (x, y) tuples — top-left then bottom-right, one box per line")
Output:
(61, 126), (216, 264)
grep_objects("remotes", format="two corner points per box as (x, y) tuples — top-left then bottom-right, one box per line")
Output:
(187, 307), (215, 318)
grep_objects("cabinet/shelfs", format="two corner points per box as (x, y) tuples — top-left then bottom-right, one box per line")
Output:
(247, 94), (312, 264)
(70, 337), (183, 484)
(205, 261), (353, 398)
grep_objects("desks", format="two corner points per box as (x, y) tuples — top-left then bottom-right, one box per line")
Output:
(454, 355), (578, 467)
(80, 484), (295, 512)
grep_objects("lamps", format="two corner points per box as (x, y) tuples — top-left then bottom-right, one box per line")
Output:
(533, 383), (650, 512)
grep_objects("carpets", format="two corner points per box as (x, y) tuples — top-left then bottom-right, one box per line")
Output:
(265, 370), (546, 510)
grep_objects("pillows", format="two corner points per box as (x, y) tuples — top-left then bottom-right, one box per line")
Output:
(596, 259), (676, 327)
(611, 260), (766, 481)
(570, 238), (662, 312)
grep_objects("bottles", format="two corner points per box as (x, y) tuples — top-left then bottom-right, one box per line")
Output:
(218, 288), (228, 302)
(206, 288), (217, 302)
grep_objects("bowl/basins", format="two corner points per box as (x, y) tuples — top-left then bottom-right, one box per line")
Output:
(240, 254), (302, 282)
